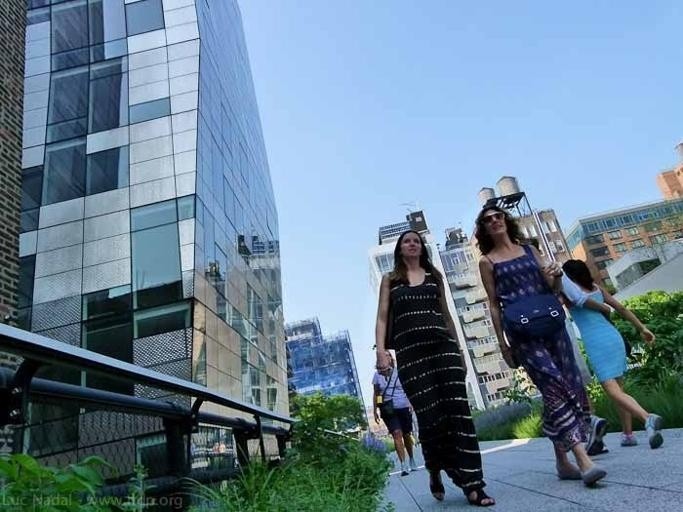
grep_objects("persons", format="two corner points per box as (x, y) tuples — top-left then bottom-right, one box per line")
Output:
(526, 235), (611, 457)
(371, 231), (498, 507)
(472, 205), (610, 489)
(372, 347), (423, 476)
(559, 257), (666, 450)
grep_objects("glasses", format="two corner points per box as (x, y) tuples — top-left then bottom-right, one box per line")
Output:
(481, 213), (506, 224)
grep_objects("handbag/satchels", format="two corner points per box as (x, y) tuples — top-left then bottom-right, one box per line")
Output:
(380, 401), (394, 417)
(503, 292), (566, 343)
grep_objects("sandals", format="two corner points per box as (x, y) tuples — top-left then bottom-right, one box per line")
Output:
(465, 489), (496, 507)
(429, 472), (446, 501)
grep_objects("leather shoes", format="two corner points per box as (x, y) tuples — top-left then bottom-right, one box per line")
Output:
(580, 464), (607, 486)
(557, 466), (582, 480)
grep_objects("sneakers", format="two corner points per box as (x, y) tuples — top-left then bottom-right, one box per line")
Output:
(645, 412), (663, 448)
(620, 435), (636, 445)
(586, 415), (609, 458)
(410, 457), (417, 470)
(401, 462), (408, 475)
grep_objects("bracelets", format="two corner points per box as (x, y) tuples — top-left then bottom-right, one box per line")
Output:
(554, 271), (564, 280)
